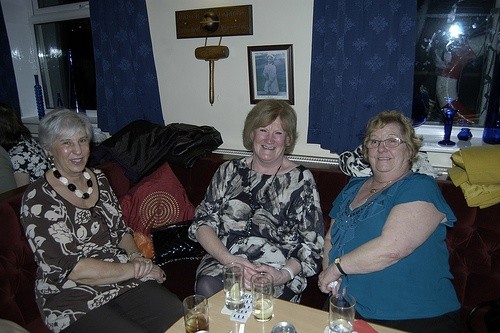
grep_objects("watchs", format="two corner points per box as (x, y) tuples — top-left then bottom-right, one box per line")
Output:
(334, 257), (346, 276)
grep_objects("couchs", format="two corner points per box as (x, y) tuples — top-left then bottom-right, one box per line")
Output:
(1, 149), (499, 333)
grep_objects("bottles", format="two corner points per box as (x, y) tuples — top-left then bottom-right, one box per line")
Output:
(482, 44), (500, 144)
(57, 92), (63, 108)
(457, 128), (472, 141)
(34, 74), (45, 120)
(66, 48), (85, 115)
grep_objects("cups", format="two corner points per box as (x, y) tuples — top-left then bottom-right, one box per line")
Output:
(329, 293), (356, 333)
(250, 271), (274, 323)
(437, 107), (456, 146)
(182, 294), (209, 333)
(222, 261), (246, 310)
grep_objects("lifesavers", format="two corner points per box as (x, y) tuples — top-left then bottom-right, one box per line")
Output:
(436, 32), (500, 128)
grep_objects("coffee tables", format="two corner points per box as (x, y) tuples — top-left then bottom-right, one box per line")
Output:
(161, 288), (407, 333)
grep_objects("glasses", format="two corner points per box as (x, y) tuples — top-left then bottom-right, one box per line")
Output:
(365, 137), (407, 151)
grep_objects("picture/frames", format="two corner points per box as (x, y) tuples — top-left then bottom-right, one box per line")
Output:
(248, 44), (293, 106)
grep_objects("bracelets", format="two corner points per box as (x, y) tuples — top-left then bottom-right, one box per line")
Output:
(282, 266), (294, 280)
(129, 251), (145, 258)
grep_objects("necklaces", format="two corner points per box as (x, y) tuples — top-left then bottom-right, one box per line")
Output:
(246, 157), (281, 235)
(370, 178), (391, 193)
(50, 162), (93, 199)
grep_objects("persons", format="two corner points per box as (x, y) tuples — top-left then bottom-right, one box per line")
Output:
(0, 105), (54, 198)
(188, 99), (324, 306)
(318, 110), (466, 333)
(21, 108), (184, 333)
(263, 53), (278, 94)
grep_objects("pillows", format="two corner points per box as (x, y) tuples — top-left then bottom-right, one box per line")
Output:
(116, 162), (196, 236)
(148, 223), (203, 266)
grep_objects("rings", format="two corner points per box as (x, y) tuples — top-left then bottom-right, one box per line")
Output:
(145, 259), (149, 263)
